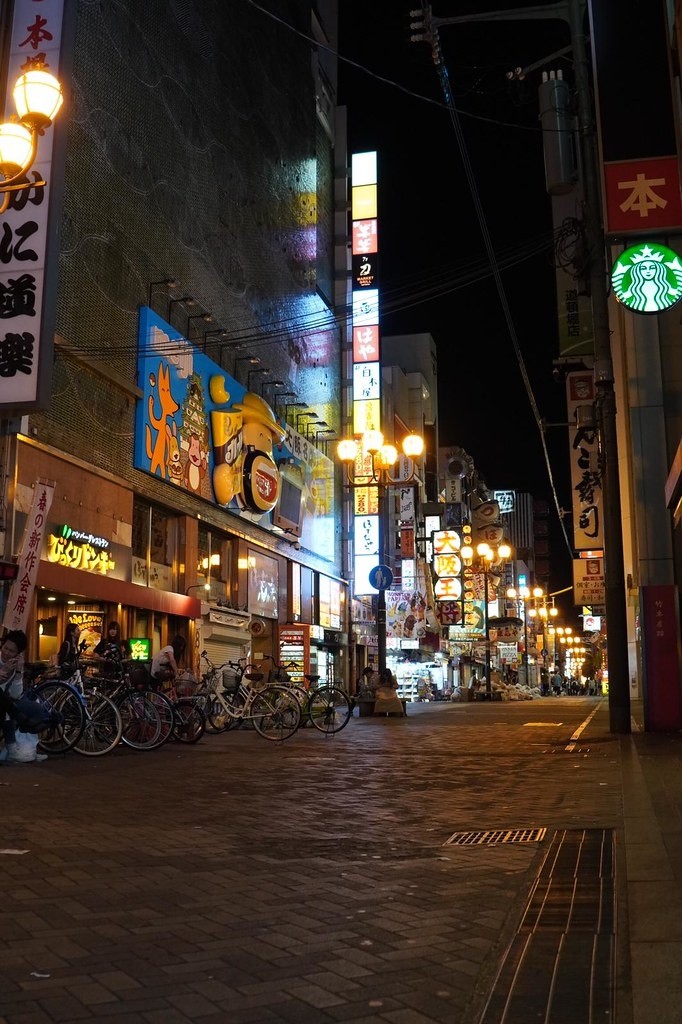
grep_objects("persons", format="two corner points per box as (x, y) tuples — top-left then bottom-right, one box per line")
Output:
(93, 622), (132, 743)
(467, 669), (517, 701)
(541, 668), (601, 696)
(148, 635), (186, 692)
(0, 630), (49, 764)
(346, 667), (376, 717)
(57, 624), (83, 687)
(373, 669), (404, 717)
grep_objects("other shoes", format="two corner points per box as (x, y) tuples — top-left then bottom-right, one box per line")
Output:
(6, 747), (34, 761)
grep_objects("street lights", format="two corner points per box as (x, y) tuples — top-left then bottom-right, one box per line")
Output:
(506, 587), (586, 697)
(334, 425), (426, 716)
(460, 544), (510, 699)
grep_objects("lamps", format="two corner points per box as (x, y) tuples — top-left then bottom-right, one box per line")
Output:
(0, 114), (34, 214)
(0, 63), (63, 186)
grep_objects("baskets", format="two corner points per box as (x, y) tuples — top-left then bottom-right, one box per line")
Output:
(268, 670), (291, 683)
(222, 670), (240, 688)
(175, 679), (197, 697)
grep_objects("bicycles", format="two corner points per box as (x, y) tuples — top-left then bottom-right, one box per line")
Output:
(3, 662), (82, 755)
(136, 661), (208, 746)
(37, 652), (124, 758)
(262, 654), (352, 738)
(199, 653), (303, 741)
(90, 652), (174, 752)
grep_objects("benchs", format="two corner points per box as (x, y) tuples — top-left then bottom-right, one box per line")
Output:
(474, 690), (504, 701)
(352, 697), (411, 718)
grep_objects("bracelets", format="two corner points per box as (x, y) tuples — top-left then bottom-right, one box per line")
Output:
(16, 670), (21, 674)
